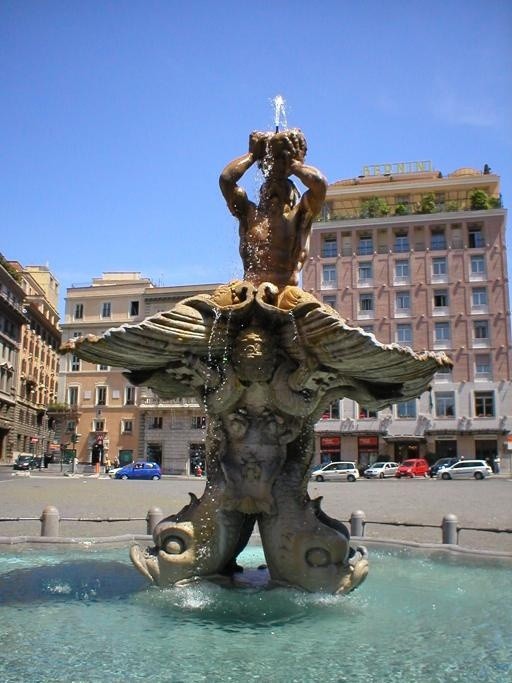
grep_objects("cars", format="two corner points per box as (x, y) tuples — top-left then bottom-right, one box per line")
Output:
(108, 461), (162, 480)
(310, 458), (492, 482)
(14, 452), (54, 469)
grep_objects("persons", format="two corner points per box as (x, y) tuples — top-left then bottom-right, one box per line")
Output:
(103, 456), (120, 473)
(485, 455), (501, 473)
(219, 126), (327, 292)
